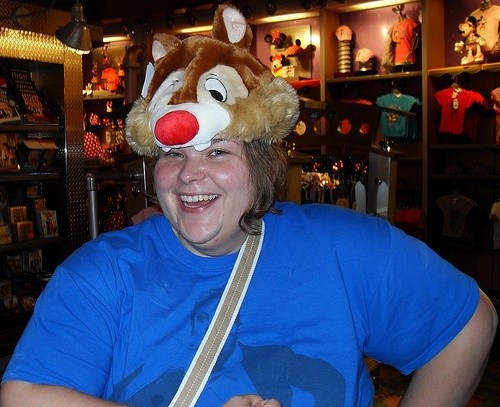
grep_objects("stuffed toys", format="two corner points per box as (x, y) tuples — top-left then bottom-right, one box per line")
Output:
(124, 1), (300, 155)
(455, 14), (486, 65)
(264, 29), (304, 78)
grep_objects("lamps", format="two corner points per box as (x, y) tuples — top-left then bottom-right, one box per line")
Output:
(11, 0), (93, 52)
(117, 0), (345, 41)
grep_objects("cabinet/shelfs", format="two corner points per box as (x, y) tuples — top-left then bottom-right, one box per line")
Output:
(0, 26), (90, 324)
(85, 0), (500, 295)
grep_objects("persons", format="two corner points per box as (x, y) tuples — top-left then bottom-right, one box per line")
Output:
(0, 118), (498, 407)
(467, 0), (500, 63)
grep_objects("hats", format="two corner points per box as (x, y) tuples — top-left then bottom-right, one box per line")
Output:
(124, 3), (300, 158)
